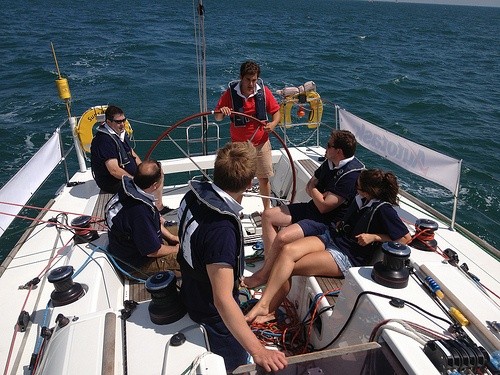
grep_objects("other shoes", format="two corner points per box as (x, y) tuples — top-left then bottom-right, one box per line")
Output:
(159, 206), (176, 216)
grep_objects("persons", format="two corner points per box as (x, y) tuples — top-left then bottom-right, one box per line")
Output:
(214, 61), (280, 209)
(90, 106), (179, 228)
(178, 142), (288, 375)
(244, 167), (412, 325)
(104, 160), (180, 281)
(241, 130), (367, 289)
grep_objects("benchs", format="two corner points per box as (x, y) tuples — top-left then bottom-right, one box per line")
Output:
(269, 153), (322, 203)
(282, 223), (347, 350)
(121, 225), (184, 303)
(84, 183), (114, 232)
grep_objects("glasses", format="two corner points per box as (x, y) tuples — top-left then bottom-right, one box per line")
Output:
(327, 142), (336, 149)
(110, 118), (127, 124)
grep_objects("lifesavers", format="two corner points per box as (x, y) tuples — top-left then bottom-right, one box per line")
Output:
(78, 106), (134, 153)
(279, 91), (324, 130)
